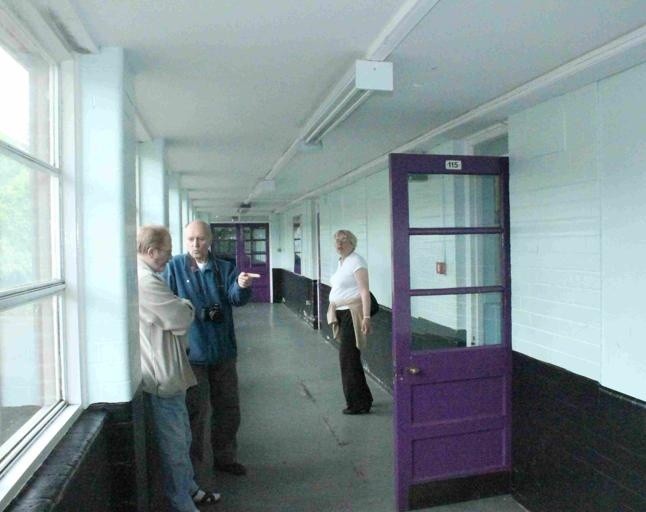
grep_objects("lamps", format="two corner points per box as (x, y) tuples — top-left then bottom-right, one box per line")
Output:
(300, 84), (377, 154)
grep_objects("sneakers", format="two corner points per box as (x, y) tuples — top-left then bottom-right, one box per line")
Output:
(214, 462), (245, 474)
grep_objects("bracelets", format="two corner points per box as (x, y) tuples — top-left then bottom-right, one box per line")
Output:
(363, 315), (372, 319)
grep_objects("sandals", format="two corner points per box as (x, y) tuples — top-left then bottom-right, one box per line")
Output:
(191, 489), (220, 505)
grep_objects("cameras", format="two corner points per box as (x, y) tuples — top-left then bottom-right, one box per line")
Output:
(201, 301), (223, 323)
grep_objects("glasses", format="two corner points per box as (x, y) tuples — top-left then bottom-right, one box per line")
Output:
(153, 245), (171, 256)
(335, 237), (349, 244)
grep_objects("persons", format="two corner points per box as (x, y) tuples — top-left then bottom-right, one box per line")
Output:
(136, 224), (223, 511)
(325, 230), (376, 415)
(161, 221), (261, 475)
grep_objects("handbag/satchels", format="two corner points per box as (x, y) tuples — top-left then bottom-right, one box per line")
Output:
(370, 291), (378, 316)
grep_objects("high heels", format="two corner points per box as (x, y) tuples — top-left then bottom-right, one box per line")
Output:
(343, 403), (371, 414)
